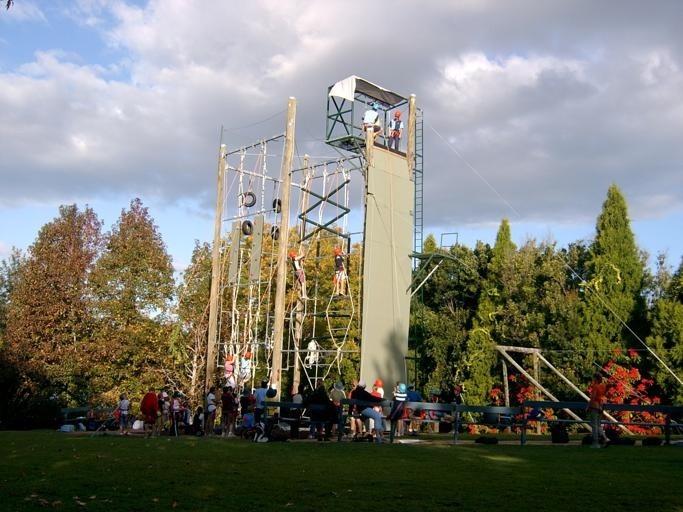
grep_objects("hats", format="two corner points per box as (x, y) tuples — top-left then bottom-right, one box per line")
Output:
(333, 381), (345, 392)
(266, 388), (277, 398)
(261, 380), (270, 385)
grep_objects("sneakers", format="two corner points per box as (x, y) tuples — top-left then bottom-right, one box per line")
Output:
(317, 437), (331, 441)
(221, 433), (237, 438)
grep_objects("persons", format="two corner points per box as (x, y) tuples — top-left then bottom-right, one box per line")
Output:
(236, 351), (251, 387)
(333, 248), (347, 297)
(304, 336), (319, 368)
(222, 355), (236, 387)
(133, 380), (268, 437)
(363, 103), (384, 143)
(291, 376), (440, 443)
(386, 111), (404, 150)
(287, 250), (307, 299)
(450, 385), (463, 404)
(585, 373), (610, 447)
(119, 394), (129, 432)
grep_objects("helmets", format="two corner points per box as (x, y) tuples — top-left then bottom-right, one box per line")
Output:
(227, 355), (232, 360)
(372, 104), (379, 110)
(395, 112), (401, 117)
(288, 250), (297, 257)
(245, 351), (251, 358)
(399, 383), (406, 394)
(334, 248), (342, 255)
(376, 378), (384, 387)
